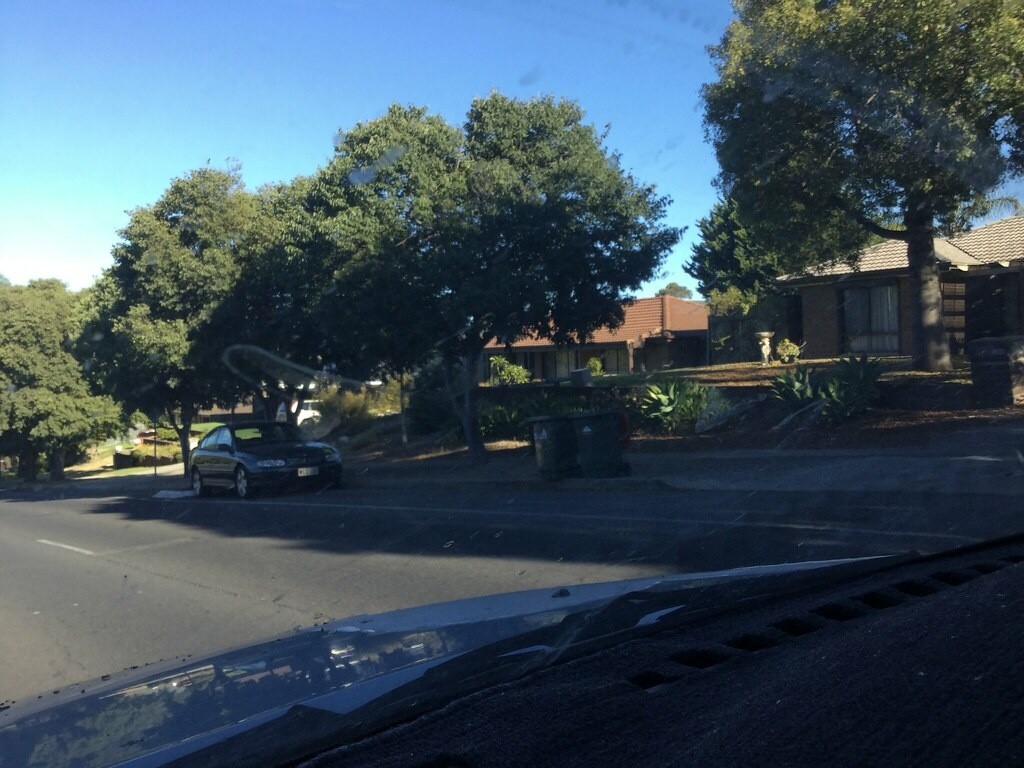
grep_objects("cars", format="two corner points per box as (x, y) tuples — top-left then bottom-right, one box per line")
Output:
(0, 532), (1024, 767)
(188, 420), (342, 497)
(134, 427), (161, 445)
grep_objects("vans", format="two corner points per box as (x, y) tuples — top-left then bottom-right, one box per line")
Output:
(276, 399), (324, 429)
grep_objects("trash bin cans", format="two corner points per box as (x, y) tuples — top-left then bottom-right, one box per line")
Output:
(527, 414), (577, 481)
(567, 411), (623, 481)
